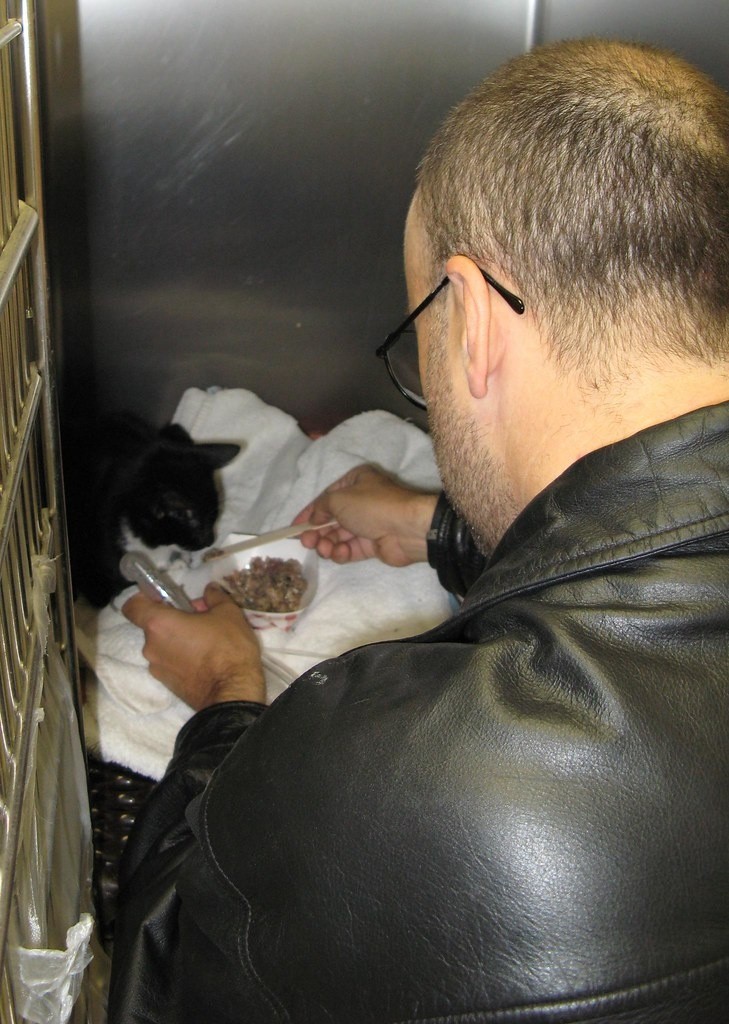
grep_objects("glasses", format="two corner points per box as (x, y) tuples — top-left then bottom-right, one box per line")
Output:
(375, 270), (524, 411)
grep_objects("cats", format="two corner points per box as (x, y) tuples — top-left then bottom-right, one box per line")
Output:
(60, 406), (242, 611)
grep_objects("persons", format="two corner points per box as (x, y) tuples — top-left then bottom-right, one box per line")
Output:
(106, 36), (729, 1024)
